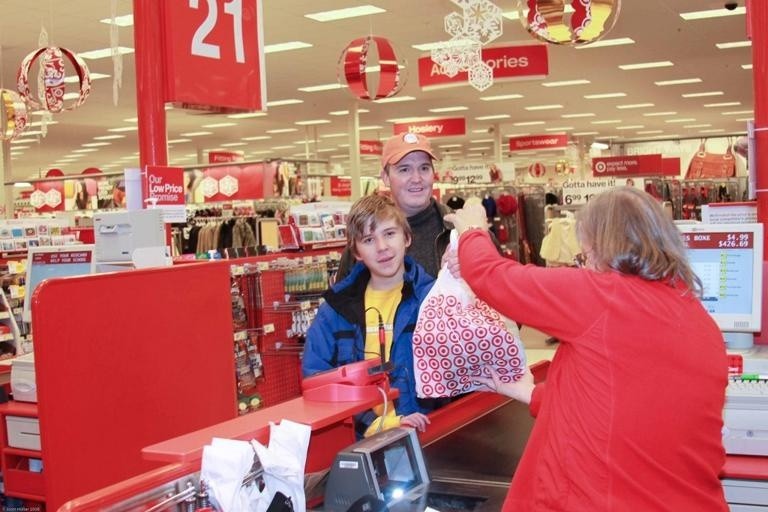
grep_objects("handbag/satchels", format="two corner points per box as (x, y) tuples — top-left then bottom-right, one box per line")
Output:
(685, 156), (736, 179)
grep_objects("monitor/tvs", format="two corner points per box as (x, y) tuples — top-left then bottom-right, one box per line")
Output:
(674, 223), (763, 357)
(21, 244), (96, 322)
(93, 208), (165, 262)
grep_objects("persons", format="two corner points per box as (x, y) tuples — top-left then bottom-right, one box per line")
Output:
(301, 191), (438, 443)
(335, 131), (523, 406)
(444, 186), (729, 512)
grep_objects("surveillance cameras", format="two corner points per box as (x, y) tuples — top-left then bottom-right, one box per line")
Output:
(724, 0), (737, 10)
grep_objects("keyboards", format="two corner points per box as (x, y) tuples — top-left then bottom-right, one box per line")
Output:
(723, 378), (768, 397)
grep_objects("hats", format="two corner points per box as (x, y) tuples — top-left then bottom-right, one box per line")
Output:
(382, 132), (438, 169)
